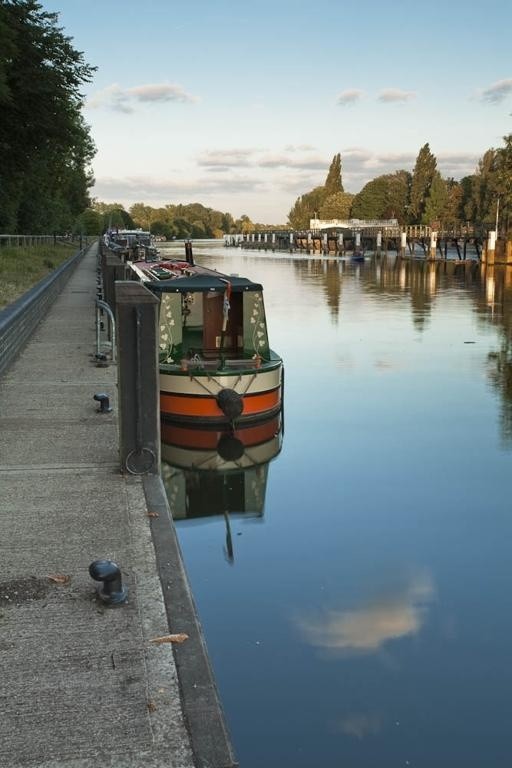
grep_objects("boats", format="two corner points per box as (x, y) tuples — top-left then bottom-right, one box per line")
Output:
(349, 253), (365, 263)
(156, 460), (274, 565)
(122, 242), (286, 467)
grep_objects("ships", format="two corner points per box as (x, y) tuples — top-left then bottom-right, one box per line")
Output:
(100, 223), (162, 264)
